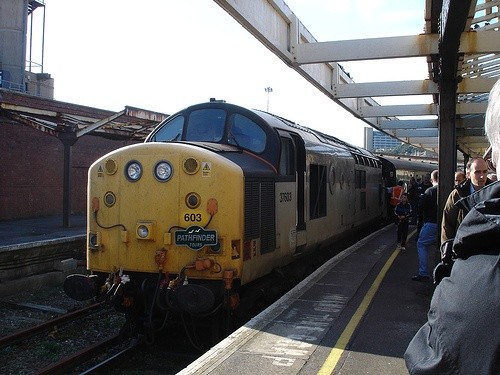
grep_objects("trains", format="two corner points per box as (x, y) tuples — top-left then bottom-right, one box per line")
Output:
(64, 98), (438, 324)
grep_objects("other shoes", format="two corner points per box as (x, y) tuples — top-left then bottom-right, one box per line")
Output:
(402, 247), (406, 250)
(411, 275), (431, 282)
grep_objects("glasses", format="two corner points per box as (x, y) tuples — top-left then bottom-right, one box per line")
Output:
(483, 145), (496, 173)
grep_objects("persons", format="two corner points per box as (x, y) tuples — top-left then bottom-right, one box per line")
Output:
(412, 169), (439, 281)
(403, 76), (500, 375)
(454, 172), (466, 187)
(441, 157), (496, 267)
(491, 175), (497, 183)
(390, 174), (432, 252)
